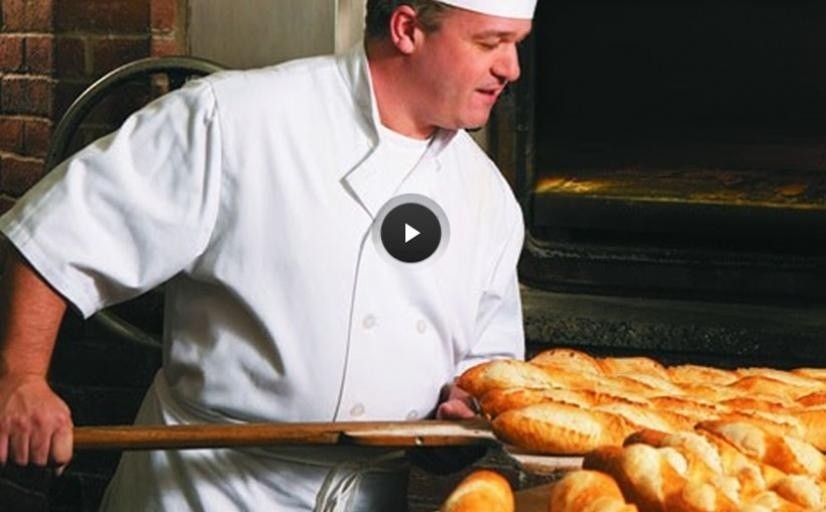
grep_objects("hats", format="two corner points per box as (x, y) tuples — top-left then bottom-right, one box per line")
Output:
(434, 0), (537, 19)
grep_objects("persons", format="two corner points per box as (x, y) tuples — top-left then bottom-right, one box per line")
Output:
(1, 1), (542, 512)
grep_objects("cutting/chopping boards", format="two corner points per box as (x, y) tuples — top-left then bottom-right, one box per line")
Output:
(341, 398), (587, 474)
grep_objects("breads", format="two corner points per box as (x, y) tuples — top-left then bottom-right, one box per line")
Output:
(438, 348), (826, 511)
